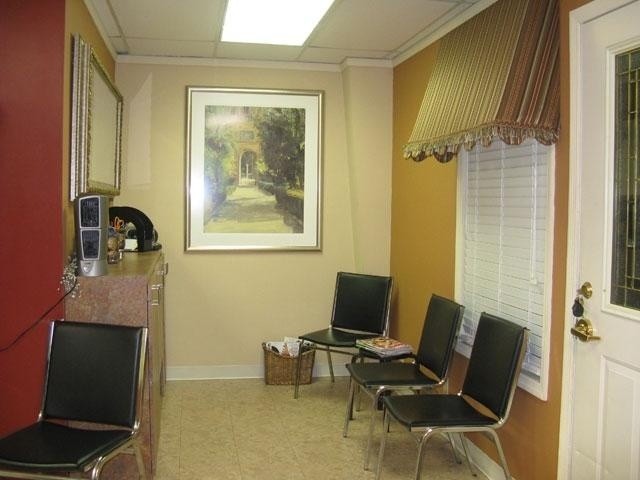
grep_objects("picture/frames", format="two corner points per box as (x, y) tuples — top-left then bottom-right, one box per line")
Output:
(185, 85), (323, 252)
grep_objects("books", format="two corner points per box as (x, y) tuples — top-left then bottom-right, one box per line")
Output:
(356, 337), (414, 358)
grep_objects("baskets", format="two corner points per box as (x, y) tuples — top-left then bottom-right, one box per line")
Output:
(262, 341), (317, 385)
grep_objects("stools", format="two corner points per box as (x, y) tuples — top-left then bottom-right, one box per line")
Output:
(344, 344), (420, 432)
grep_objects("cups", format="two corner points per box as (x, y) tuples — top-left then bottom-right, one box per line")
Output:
(108, 228), (127, 264)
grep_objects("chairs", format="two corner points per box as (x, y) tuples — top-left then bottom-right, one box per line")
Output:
(342, 291), (485, 473)
(373, 311), (531, 480)
(296, 267), (394, 390)
(2, 318), (155, 480)
